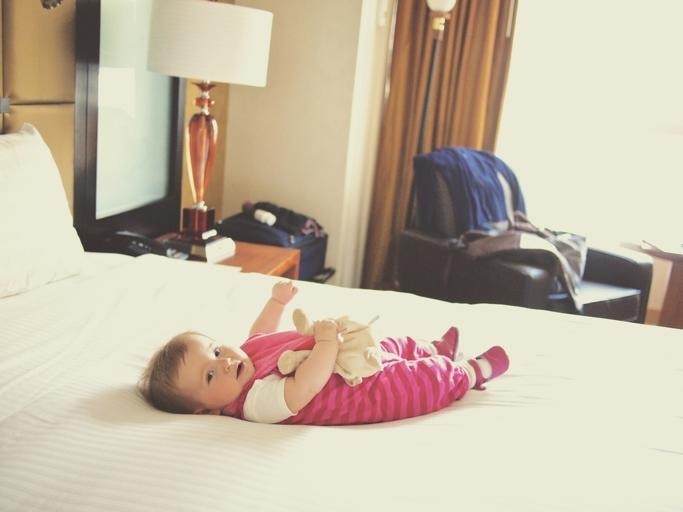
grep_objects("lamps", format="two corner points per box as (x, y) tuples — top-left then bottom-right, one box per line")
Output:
(146, 2), (275, 240)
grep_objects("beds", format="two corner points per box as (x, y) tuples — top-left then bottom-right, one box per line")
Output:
(0, 252), (681, 509)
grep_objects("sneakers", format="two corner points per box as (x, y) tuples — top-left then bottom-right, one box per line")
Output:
(468, 344), (510, 391)
(431, 326), (459, 359)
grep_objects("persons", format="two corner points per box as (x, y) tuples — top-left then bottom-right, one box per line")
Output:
(135, 281), (511, 425)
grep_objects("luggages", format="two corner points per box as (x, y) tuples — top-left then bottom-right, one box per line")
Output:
(219, 210), (331, 283)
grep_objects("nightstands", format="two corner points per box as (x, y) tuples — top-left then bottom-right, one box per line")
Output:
(151, 233), (303, 283)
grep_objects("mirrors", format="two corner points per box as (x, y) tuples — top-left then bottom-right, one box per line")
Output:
(72, 2), (188, 257)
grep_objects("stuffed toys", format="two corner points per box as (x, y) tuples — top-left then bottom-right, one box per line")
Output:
(278, 307), (382, 387)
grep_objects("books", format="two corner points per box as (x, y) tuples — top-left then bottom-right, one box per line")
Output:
(153, 230), (236, 262)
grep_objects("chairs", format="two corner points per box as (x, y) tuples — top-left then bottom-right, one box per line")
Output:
(398, 155), (655, 324)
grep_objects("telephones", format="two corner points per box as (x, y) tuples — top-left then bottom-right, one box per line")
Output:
(102, 229), (189, 262)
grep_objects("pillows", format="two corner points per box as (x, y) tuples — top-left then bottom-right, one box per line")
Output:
(0, 120), (88, 302)
